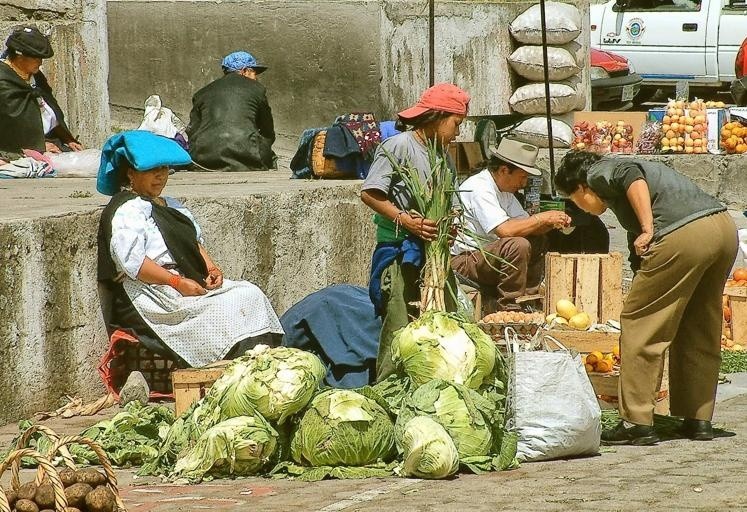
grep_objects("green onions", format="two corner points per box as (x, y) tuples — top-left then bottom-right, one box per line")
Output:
(377, 130), (516, 314)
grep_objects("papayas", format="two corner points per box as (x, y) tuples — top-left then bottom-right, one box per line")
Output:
(547, 298), (591, 332)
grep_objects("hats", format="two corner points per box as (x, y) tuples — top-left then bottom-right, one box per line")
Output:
(6, 27), (54, 58)
(397, 83), (471, 125)
(488, 137), (542, 176)
(221, 51), (267, 74)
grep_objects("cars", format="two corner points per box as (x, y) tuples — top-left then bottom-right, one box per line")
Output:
(588, 49), (642, 111)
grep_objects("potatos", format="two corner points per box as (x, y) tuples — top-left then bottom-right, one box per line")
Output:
(5, 465), (118, 512)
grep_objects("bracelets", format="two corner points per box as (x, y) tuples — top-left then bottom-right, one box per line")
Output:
(169, 274), (181, 288)
(531, 213), (540, 230)
(207, 266), (219, 274)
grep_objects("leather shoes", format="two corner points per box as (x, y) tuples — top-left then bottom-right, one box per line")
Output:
(600, 420), (659, 446)
(680, 419), (713, 440)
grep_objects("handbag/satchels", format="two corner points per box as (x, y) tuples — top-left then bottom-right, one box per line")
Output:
(503, 327), (601, 462)
(729, 76), (746, 107)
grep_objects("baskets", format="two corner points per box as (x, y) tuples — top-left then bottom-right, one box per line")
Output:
(311, 130), (353, 177)
(1, 425), (128, 512)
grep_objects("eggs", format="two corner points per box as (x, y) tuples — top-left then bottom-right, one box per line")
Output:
(482, 311), (539, 341)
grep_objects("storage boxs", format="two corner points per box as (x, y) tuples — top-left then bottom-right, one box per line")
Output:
(573, 112), (648, 152)
(649, 109), (724, 154)
(729, 107), (747, 130)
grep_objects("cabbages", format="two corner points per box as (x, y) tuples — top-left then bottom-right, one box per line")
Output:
(281, 388), (395, 478)
(394, 379), (519, 479)
(210, 343), (326, 417)
(386, 314), (496, 393)
(155, 416), (279, 477)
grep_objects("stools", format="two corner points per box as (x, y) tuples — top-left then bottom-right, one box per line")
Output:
(454, 269), (497, 322)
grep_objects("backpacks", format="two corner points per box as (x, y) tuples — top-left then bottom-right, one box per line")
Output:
(136, 94), (189, 144)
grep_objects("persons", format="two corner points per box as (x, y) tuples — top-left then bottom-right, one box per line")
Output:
(184, 52), (277, 170)
(556, 149), (738, 445)
(96, 131), (285, 371)
(448, 138), (570, 310)
(0, 26), (83, 164)
(359, 82), (465, 323)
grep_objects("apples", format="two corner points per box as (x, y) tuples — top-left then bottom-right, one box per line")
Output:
(582, 346), (619, 373)
(573, 98), (747, 154)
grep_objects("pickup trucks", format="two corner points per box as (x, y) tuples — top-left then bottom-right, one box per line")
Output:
(588, 0), (747, 105)
